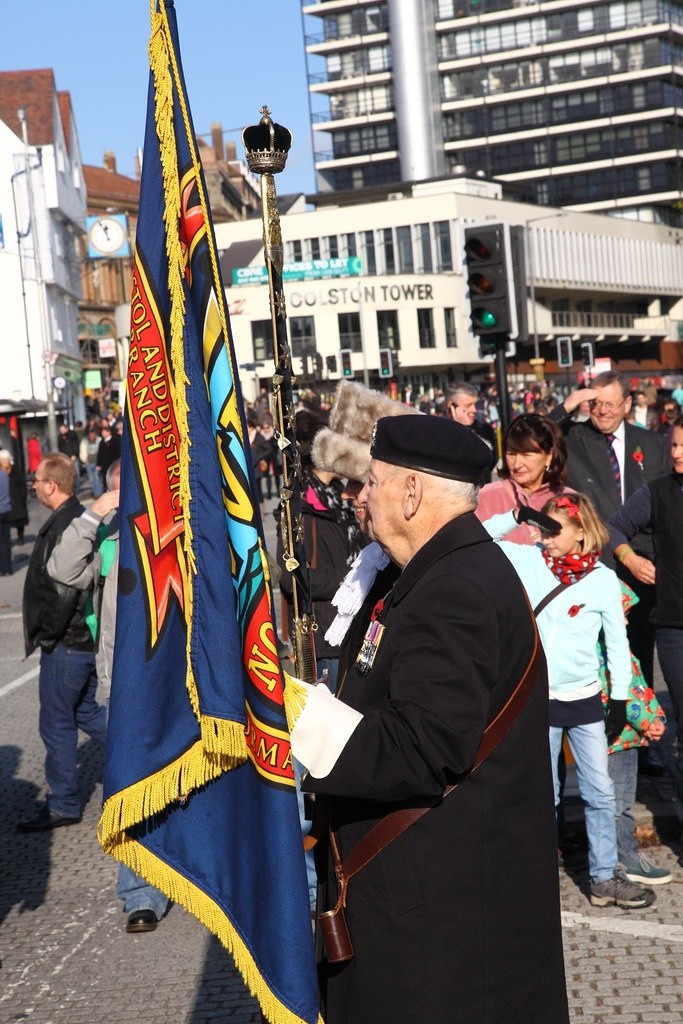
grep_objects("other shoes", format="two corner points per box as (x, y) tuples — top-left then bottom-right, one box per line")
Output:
(18, 538), (24, 545)
(0, 569), (13, 576)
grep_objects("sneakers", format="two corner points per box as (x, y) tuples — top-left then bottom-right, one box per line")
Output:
(620, 853), (672, 884)
(588, 863), (656, 907)
(557, 847), (586, 870)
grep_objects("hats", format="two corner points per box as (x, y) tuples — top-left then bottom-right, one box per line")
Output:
(370, 415), (495, 482)
(311, 378), (493, 481)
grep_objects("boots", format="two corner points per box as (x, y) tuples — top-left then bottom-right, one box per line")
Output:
(275, 476), (283, 498)
(266, 479), (272, 500)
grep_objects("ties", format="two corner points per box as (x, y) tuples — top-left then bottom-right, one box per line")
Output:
(604, 433), (621, 506)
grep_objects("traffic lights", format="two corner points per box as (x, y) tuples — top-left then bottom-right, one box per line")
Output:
(557, 337), (572, 367)
(340, 350), (354, 378)
(462, 220), (514, 335)
(378, 349), (393, 378)
(581, 343), (592, 367)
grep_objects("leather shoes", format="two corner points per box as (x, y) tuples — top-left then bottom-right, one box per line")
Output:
(126, 909), (157, 932)
(17, 807), (81, 832)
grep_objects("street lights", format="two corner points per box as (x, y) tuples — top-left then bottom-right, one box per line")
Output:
(526, 212), (568, 358)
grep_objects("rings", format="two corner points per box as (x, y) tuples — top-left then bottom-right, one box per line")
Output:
(643, 576), (648, 581)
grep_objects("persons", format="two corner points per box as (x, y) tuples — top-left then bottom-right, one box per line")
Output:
(0, 368), (682, 907)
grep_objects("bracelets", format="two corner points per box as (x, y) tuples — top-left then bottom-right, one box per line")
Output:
(619, 548), (631, 562)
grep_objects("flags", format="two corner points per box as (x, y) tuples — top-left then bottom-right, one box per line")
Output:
(98, 1), (333, 1023)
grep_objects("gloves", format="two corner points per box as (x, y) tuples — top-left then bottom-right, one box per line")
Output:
(605, 699), (627, 746)
(517, 506), (562, 533)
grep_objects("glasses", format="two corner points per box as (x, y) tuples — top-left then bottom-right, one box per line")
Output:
(663, 409), (674, 414)
(32, 477), (48, 484)
(589, 396), (625, 411)
